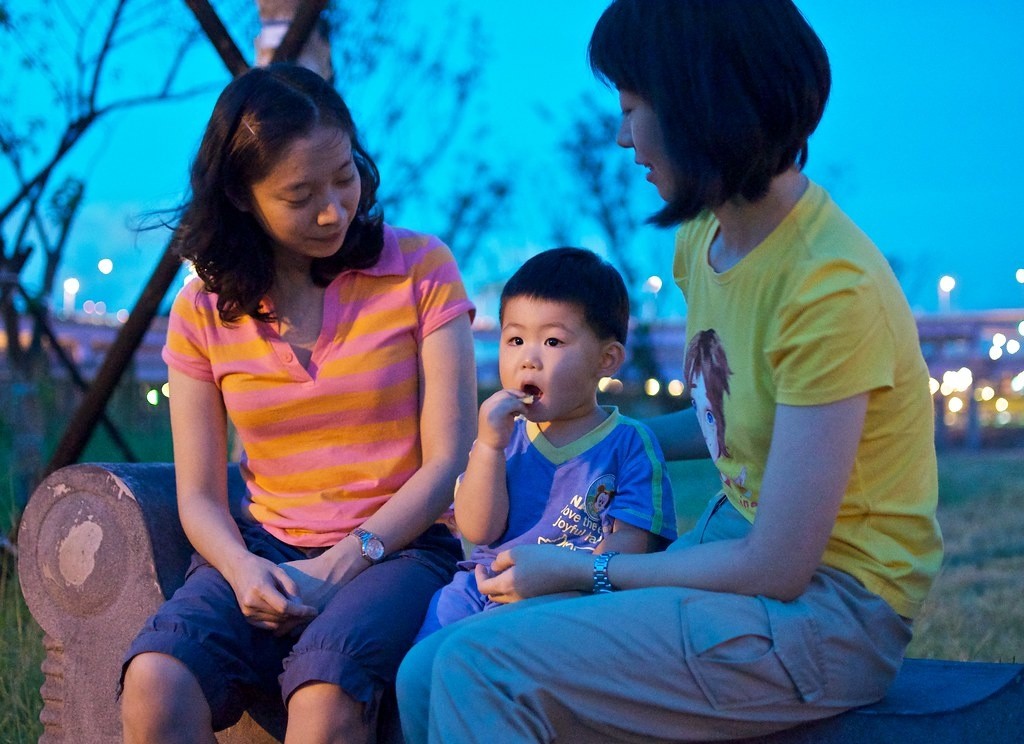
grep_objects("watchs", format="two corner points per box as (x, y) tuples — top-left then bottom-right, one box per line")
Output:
(592, 552), (618, 594)
(349, 528), (387, 564)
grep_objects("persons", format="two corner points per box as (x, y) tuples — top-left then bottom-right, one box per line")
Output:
(397, 0), (943, 743)
(116, 62), (479, 743)
(413, 244), (675, 647)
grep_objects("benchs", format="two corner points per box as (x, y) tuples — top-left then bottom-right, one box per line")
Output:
(15, 460), (1024, 744)
